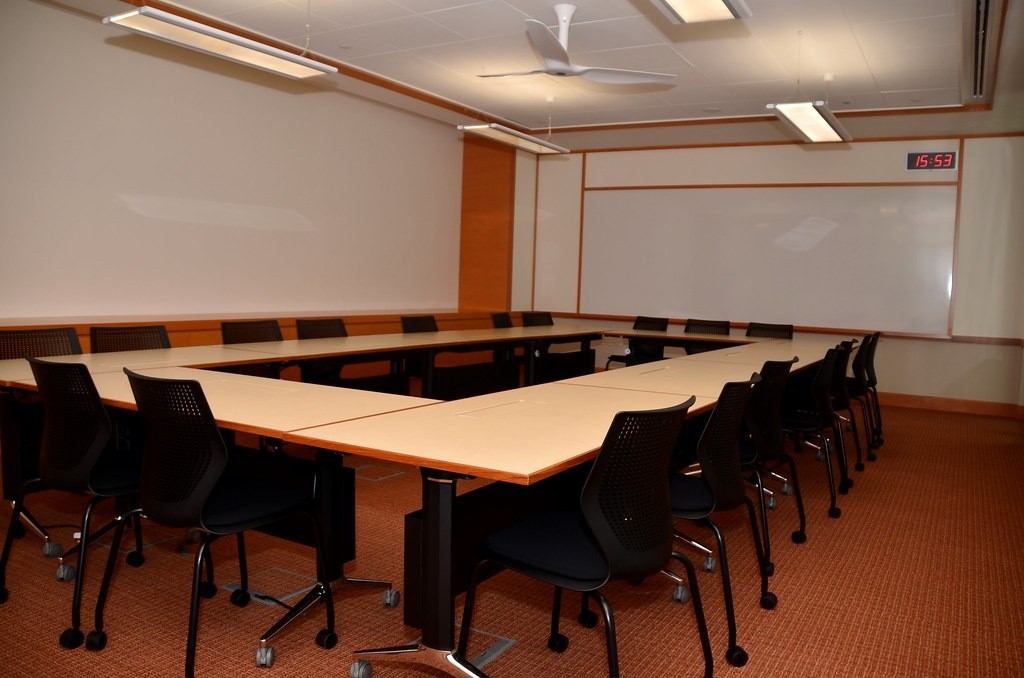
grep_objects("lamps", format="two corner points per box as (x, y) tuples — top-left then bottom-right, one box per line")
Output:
(457, 97), (571, 155)
(766, 31), (855, 145)
(102, 0), (348, 80)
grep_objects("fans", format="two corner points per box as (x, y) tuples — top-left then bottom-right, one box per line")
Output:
(473, 4), (677, 87)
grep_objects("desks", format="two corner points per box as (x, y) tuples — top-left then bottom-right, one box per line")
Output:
(0, 326), (862, 604)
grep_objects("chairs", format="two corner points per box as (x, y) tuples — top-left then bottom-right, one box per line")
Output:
(0, 327), (83, 360)
(89, 325), (174, 354)
(0, 355), (218, 648)
(578, 371), (778, 667)
(605, 316), (673, 371)
(739, 330), (885, 576)
(684, 318), (730, 355)
(746, 322), (793, 339)
(491, 313), (513, 328)
(86, 367), (339, 678)
(400, 316), (441, 377)
(295, 318), (349, 385)
(521, 312), (555, 327)
(220, 320), (284, 344)
(455, 396), (714, 677)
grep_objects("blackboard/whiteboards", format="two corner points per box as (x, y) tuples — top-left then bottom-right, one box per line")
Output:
(577, 181), (962, 339)
